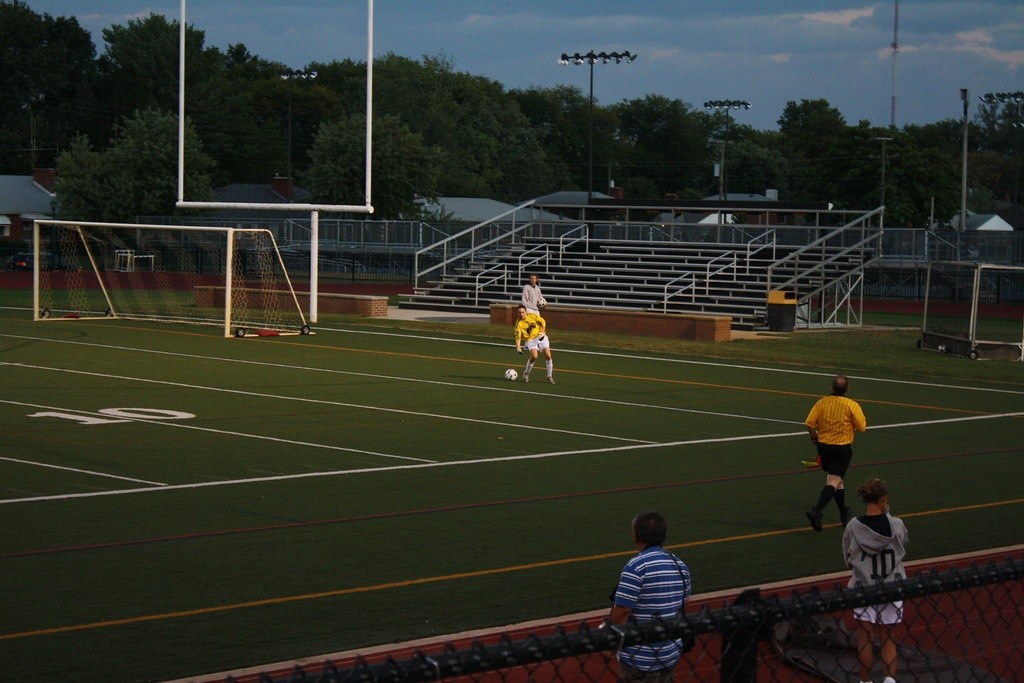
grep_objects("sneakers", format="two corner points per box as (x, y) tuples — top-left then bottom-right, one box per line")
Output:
(546, 376), (555, 384)
(840, 508), (852, 527)
(806, 505), (823, 532)
(523, 371), (528, 382)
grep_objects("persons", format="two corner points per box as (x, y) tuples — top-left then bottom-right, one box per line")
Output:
(521, 275), (544, 317)
(803, 374), (866, 532)
(608, 512), (691, 683)
(841, 478), (909, 683)
(514, 305), (556, 384)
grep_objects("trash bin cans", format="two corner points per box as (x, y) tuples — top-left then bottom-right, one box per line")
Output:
(767, 290), (797, 331)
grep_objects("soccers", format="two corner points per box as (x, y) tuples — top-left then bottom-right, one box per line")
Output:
(537, 298), (546, 307)
(505, 369), (518, 381)
(938, 344), (948, 353)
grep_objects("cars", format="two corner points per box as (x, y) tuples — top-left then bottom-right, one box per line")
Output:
(6, 251), (82, 272)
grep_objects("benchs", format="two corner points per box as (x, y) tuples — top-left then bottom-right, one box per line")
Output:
(398, 232), (875, 329)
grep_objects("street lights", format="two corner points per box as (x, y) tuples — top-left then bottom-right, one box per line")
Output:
(703, 100), (752, 201)
(280, 70), (319, 203)
(557, 50), (637, 249)
